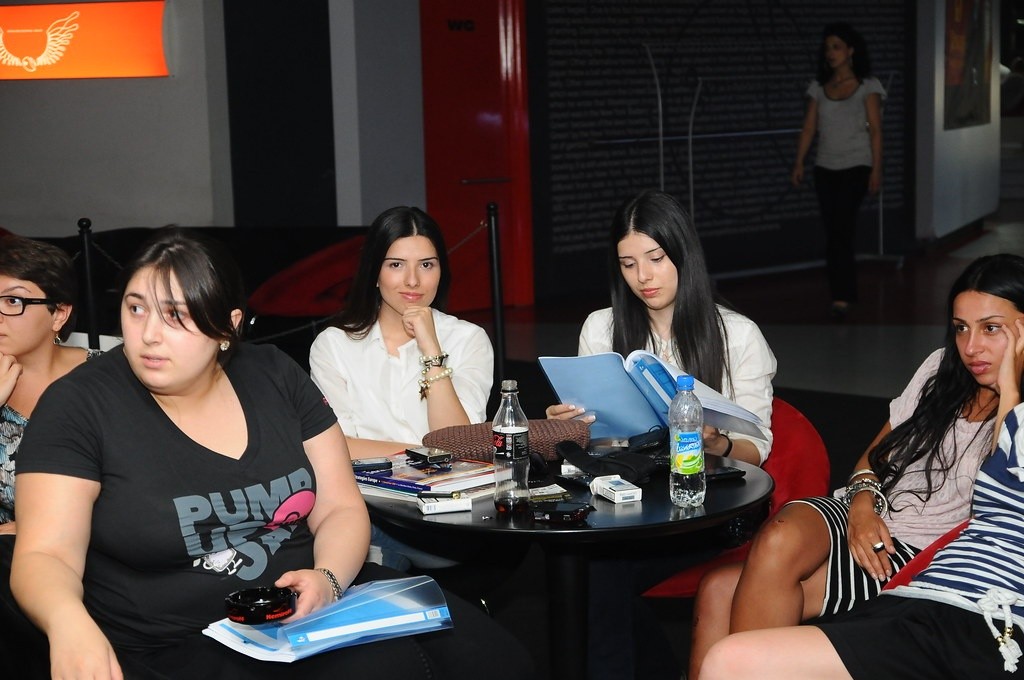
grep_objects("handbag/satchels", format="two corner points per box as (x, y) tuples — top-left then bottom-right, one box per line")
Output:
(423, 418), (590, 464)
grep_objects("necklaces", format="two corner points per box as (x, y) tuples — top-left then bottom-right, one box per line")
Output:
(651, 328), (669, 361)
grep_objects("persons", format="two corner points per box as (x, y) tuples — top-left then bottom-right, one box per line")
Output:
(0, 235), (106, 680)
(546, 190), (777, 680)
(791, 22), (888, 318)
(698, 404), (1024, 680)
(9, 229), (537, 680)
(689, 254), (1024, 680)
(309, 205), (494, 572)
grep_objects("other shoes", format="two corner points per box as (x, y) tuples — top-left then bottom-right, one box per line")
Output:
(830, 301), (848, 319)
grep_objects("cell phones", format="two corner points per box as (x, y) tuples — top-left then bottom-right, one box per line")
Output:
(351, 457), (392, 471)
(406, 447), (453, 463)
(706, 466), (745, 481)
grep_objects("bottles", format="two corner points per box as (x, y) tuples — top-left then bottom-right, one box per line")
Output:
(669, 376), (706, 505)
(493, 380), (535, 517)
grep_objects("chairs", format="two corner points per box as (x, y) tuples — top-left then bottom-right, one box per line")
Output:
(639, 394), (830, 600)
(247, 235), (366, 319)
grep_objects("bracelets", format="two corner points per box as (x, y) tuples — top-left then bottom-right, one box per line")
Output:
(317, 568), (342, 602)
(843, 469), (888, 519)
(418, 353), (453, 400)
(720, 434), (732, 457)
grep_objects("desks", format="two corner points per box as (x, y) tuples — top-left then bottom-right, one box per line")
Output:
(358, 421), (775, 680)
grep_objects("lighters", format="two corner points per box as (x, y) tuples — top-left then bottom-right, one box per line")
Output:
(417, 490), (461, 499)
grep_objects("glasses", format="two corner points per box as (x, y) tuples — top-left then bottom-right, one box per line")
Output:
(0, 293), (60, 316)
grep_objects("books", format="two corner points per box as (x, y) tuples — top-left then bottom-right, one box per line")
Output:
(539, 350), (767, 441)
(354, 450), (516, 503)
(201, 576), (453, 664)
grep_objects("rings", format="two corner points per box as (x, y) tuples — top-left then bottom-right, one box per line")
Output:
(873, 543), (884, 552)
